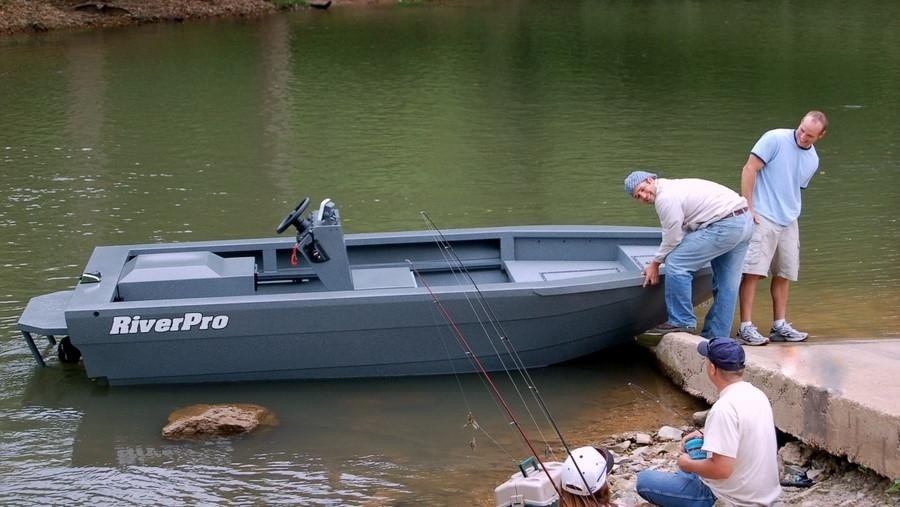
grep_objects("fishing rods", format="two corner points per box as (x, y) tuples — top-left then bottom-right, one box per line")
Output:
(404, 258), (569, 507)
(628, 382), (706, 459)
(420, 210), (600, 507)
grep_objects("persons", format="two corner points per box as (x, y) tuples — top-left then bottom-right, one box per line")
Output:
(559, 446), (624, 506)
(741, 111), (829, 345)
(623, 170), (753, 342)
(635, 338), (782, 506)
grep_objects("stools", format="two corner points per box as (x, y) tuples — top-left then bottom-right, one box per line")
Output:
(350, 265), (418, 290)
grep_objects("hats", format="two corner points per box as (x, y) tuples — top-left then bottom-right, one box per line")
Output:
(561, 445), (615, 496)
(624, 170), (657, 194)
(697, 336), (745, 370)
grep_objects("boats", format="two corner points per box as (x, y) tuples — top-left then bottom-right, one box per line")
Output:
(14, 195), (719, 393)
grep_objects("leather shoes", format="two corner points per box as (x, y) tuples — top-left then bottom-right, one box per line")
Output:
(654, 321), (695, 332)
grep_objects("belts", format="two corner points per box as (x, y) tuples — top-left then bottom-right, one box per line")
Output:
(722, 205), (748, 219)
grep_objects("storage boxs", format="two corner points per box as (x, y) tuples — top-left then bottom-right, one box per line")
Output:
(494, 457), (567, 507)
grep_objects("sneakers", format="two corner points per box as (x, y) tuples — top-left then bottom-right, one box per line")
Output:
(770, 320), (809, 342)
(735, 323), (769, 345)
